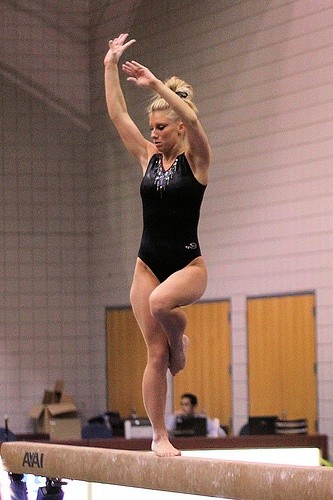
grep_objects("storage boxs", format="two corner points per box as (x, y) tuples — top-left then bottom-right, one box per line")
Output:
(28, 379), (81, 440)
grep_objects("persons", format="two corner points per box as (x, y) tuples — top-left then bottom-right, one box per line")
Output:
(167, 393), (215, 436)
(105, 33), (211, 458)
